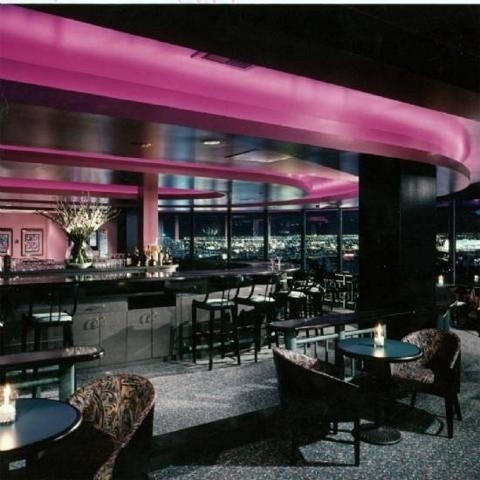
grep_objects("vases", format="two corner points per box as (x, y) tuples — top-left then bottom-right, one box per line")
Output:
(65, 240), (94, 269)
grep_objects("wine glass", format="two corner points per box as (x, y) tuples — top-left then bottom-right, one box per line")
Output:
(276, 253), (283, 265)
(268, 251), (276, 270)
(93, 254), (126, 270)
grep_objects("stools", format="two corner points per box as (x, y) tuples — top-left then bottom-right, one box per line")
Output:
(449, 301), (467, 329)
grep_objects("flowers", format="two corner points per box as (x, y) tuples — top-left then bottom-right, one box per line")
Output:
(33, 191), (122, 263)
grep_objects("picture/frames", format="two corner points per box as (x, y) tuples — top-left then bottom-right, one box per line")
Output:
(21, 228), (43, 257)
(0, 228), (13, 257)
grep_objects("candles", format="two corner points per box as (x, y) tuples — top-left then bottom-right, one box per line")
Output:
(0, 385), (16, 423)
(438, 275), (444, 286)
(374, 324), (384, 347)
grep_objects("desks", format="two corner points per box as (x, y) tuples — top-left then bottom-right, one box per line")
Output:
(436, 283), (456, 300)
(0, 397), (83, 480)
(266, 310), (411, 349)
(336, 338), (423, 445)
(0, 345), (106, 397)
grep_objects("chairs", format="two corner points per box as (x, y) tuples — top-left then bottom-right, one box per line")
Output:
(230, 272), (355, 362)
(22, 283), (79, 380)
(28, 373), (157, 480)
(192, 275), (244, 370)
(273, 346), (359, 467)
(389, 328), (462, 439)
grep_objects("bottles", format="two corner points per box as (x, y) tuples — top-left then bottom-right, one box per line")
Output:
(0, 387), (18, 424)
(373, 322), (386, 347)
